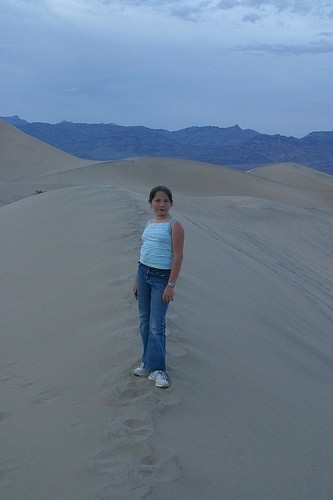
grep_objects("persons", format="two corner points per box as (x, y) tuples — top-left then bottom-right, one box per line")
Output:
(133, 186), (185, 388)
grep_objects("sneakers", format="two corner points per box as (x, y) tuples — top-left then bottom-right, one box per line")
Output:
(134, 365), (150, 377)
(147, 371), (170, 388)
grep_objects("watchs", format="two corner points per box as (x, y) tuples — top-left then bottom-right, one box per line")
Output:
(168, 282), (175, 288)
